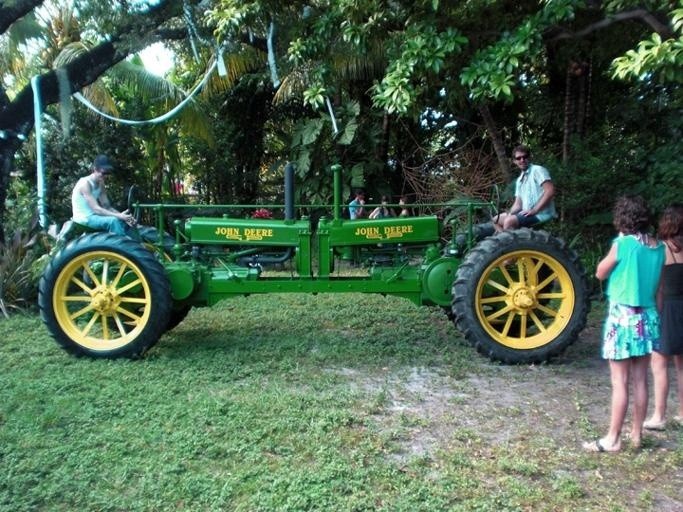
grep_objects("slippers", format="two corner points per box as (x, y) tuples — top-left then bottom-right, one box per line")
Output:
(589, 437), (622, 453)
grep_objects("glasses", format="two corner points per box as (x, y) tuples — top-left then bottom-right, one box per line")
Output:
(515, 154), (529, 160)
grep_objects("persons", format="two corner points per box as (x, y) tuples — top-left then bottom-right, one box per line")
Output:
(643, 202), (682, 430)
(368, 194), (396, 219)
(397, 196), (411, 218)
(582, 193), (667, 453)
(493, 145), (559, 233)
(348, 189), (367, 220)
(71, 155), (143, 247)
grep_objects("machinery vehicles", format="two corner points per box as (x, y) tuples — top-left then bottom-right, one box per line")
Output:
(38, 160), (589, 364)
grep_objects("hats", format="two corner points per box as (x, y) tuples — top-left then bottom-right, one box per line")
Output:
(94, 155), (114, 170)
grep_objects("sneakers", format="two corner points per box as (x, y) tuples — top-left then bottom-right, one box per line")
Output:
(643, 419), (668, 431)
